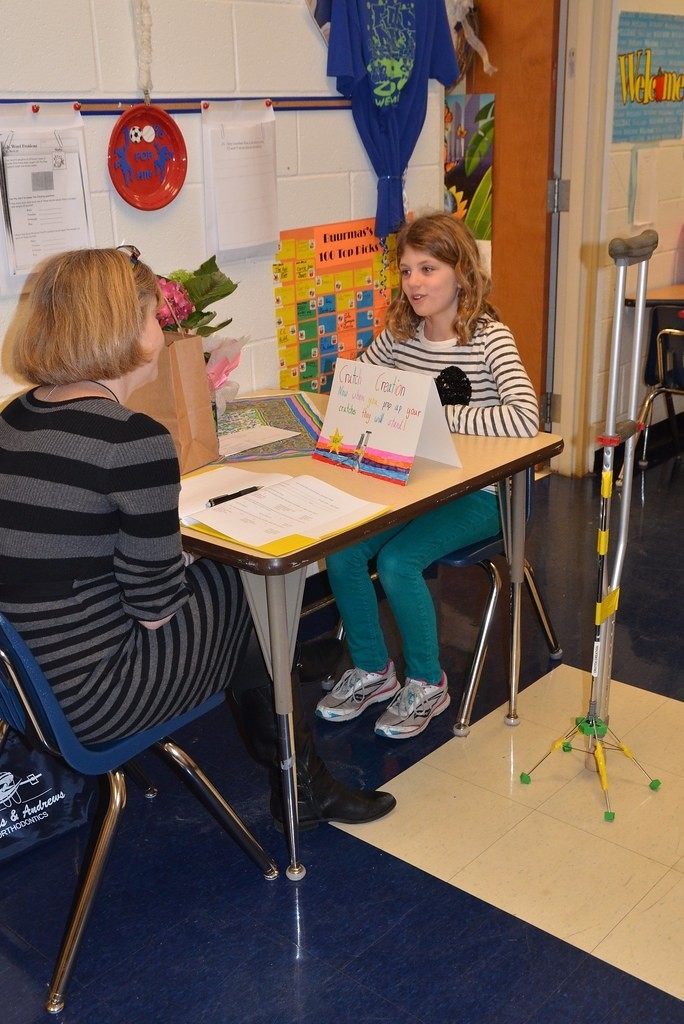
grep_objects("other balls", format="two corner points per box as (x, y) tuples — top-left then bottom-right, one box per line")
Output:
(143, 125), (155, 143)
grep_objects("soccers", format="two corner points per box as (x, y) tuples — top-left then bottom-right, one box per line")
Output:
(129, 125), (142, 144)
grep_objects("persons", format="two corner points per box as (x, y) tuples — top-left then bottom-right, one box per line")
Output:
(0, 245), (397, 832)
(314, 210), (540, 739)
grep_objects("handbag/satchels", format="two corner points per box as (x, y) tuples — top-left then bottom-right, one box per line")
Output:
(123, 297), (218, 477)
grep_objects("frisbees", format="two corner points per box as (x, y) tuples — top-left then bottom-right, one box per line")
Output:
(106, 104), (189, 212)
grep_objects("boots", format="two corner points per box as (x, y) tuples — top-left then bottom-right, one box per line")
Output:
(290, 635), (345, 684)
(227, 683), (398, 834)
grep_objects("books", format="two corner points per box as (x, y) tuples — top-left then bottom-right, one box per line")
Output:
(162, 465), (391, 558)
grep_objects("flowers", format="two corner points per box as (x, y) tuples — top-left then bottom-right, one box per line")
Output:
(155, 254), (242, 337)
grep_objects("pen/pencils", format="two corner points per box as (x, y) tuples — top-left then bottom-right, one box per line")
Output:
(205, 485), (264, 506)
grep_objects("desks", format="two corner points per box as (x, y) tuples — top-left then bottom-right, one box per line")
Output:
(625, 281), (684, 460)
(178, 390), (562, 881)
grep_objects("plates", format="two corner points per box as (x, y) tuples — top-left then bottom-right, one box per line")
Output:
(107, 105), (188, 212)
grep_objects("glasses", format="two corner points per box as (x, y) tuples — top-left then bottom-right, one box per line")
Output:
(115, 245), (140, 268)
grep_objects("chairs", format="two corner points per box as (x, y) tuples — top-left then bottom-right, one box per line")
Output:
(321, 461), (562, 736)
(1, 612), (280, 1015)
(616, 305), (684, 494)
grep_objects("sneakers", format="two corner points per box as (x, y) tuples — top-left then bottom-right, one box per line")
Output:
(374, 668), (451, 739)
(314, 659), (402, 723)
